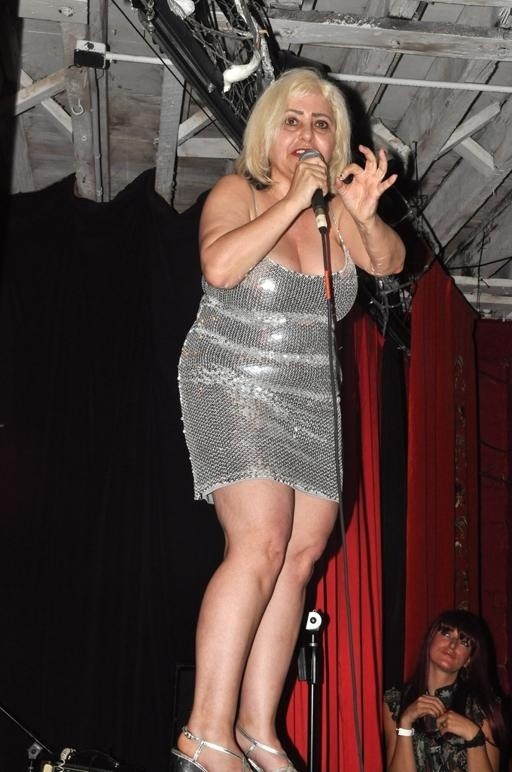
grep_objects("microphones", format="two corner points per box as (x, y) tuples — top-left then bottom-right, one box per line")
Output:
(300, 149), (330, 234)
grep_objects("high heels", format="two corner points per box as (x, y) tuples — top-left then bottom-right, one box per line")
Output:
(167, 726), (256, 771)
(234, 724), (300, 772)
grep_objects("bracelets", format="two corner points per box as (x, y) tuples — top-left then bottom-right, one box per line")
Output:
(464, 728), (485, 747)
(395, 727), (415, 736)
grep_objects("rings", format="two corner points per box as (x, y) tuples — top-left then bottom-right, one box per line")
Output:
(441, 721), (446, 727)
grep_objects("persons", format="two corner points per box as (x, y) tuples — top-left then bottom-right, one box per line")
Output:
(171, 66), (405, 772)
(384, 608), (510, 771)
